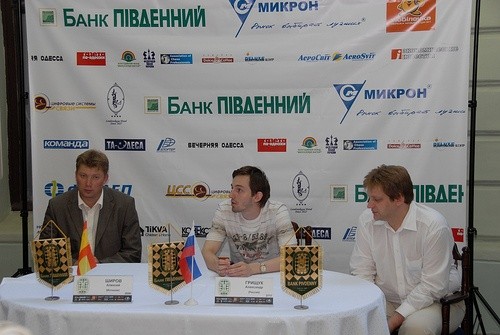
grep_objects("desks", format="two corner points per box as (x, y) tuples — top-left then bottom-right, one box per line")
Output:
(0, 263), (391, 335)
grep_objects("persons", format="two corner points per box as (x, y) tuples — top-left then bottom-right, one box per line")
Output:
(39, 149), (142, 264)
(201, 166), (297, 277)
(349, 164), (466, 335)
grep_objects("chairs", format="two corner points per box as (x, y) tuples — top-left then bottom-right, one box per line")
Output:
(440, 241), (472, 335)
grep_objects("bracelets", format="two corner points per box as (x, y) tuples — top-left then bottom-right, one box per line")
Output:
(259, 262), (266, 274)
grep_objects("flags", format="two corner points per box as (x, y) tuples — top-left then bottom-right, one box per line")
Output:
(77, 218), (96, 276)
(179, 220), (208, 284)
